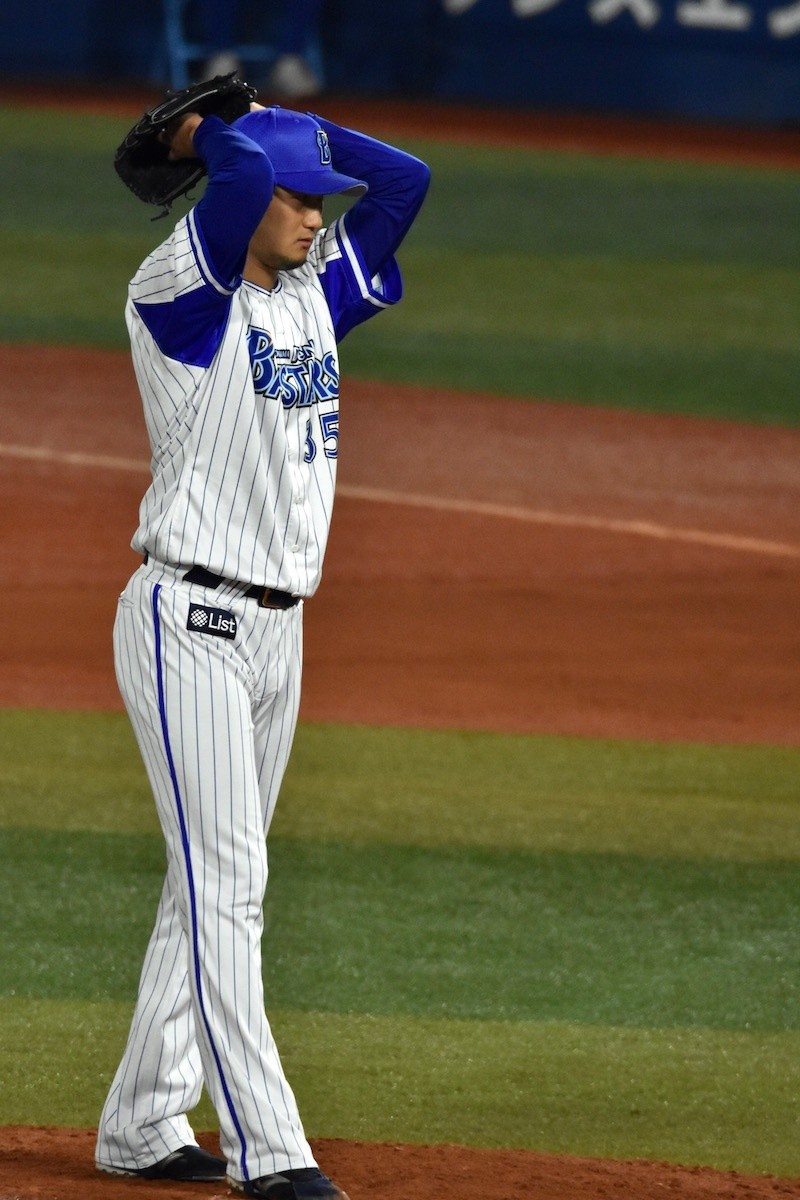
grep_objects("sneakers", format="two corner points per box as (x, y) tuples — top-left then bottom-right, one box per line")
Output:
(225, 1163), (349, 1199)
(94, 1140), (223, 1182)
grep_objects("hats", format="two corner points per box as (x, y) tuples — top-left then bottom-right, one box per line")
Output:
(231, 105), (369, 198)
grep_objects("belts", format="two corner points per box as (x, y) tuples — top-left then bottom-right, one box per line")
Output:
(143, 548), (302, 612)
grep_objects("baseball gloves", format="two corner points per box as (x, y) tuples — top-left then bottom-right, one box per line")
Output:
(111, 66), (262, 224)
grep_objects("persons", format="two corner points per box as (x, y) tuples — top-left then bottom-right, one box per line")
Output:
(92, 102), (433, 1200)
